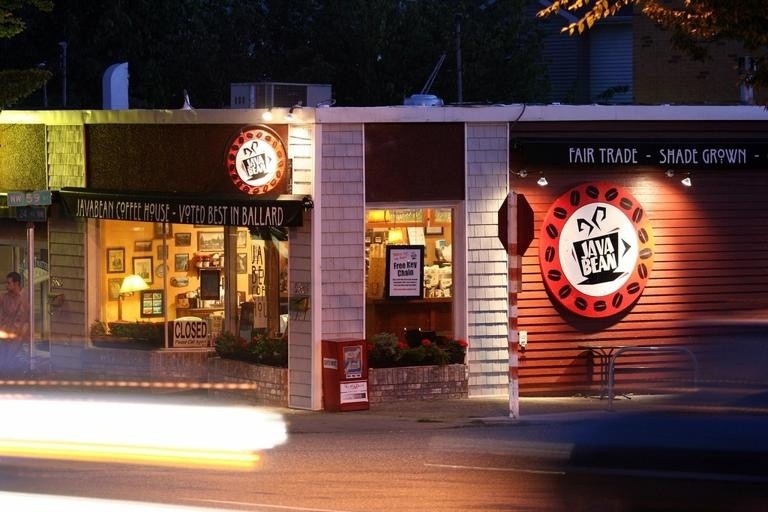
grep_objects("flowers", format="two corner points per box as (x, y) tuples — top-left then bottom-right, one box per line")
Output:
(368, 332), (468, 368)
(211, 325), (289, 369)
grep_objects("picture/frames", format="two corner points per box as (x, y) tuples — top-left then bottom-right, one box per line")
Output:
(106, 222), (249, 319)
(384, 244), (425, 301)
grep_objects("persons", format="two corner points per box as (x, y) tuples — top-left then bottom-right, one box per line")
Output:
(0, 271), (29, 375)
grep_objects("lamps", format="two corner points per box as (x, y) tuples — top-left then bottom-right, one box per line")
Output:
(668, 170), (694, 189)
(512, 168), (551, 187)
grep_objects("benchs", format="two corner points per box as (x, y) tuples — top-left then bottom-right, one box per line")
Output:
(578, 342), (703, 412)
